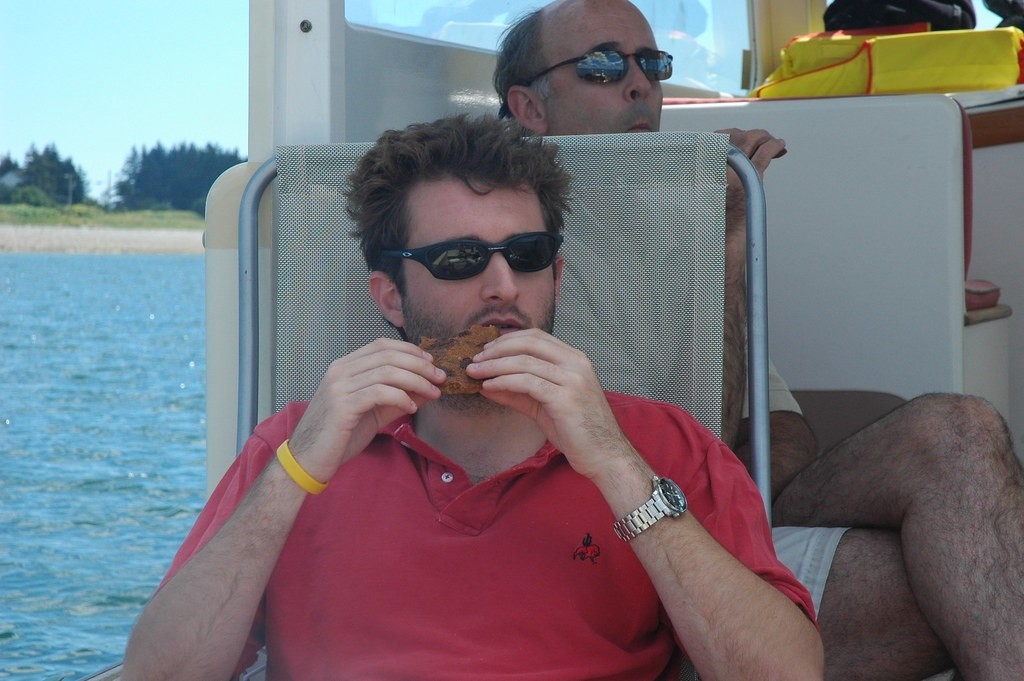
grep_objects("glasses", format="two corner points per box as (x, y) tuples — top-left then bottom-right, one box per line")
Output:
(381, 232), (564, 280)
(523, 47), (674, 84)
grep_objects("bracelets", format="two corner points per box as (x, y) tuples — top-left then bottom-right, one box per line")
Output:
(277, 440), (329, 494)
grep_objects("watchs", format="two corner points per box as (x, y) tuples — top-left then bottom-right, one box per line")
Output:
(614, 475), (688, 541)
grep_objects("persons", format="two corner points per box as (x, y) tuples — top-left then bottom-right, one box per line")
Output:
(492, 0), (1024, 681)
(125, 110), (824, 681)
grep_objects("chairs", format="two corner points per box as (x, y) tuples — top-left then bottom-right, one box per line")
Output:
(237, 131), (773, 544)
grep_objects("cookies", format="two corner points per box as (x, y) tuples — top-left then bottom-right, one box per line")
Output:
(751, 144), (788, 158)
(418, 325), (502, 394)
